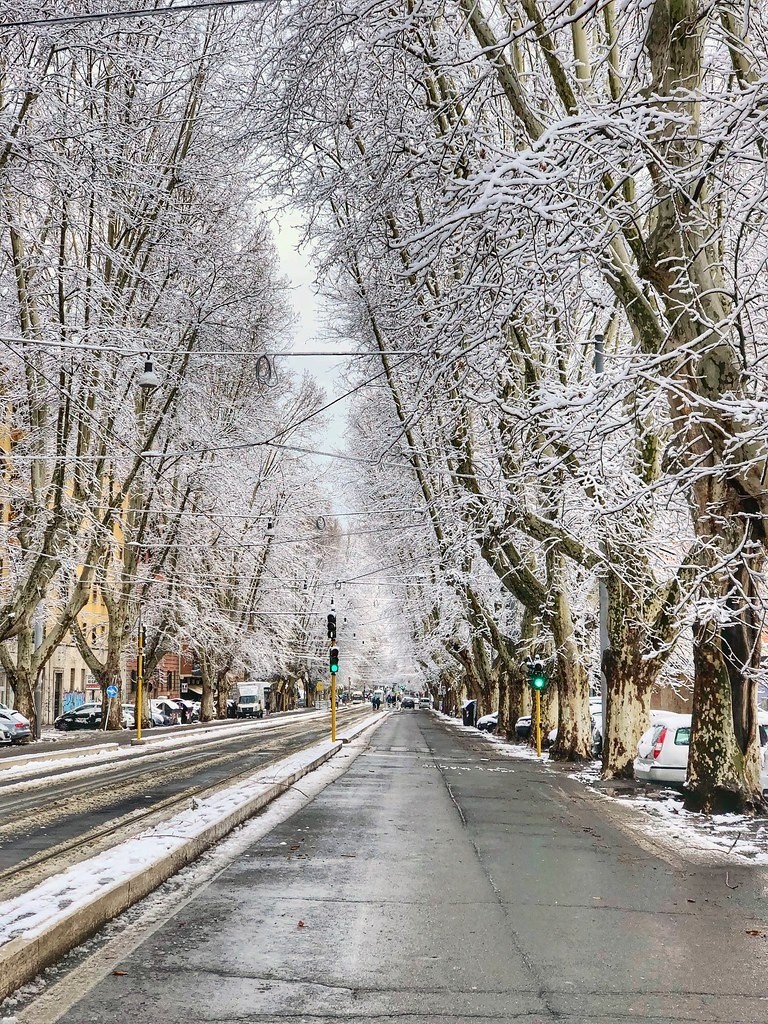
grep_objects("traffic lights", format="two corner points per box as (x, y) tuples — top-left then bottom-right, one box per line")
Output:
(533, 660), (546, 690)
(329, 647), (339, 673)
(524, 662), (535, 685)
(327, 613), (336, 638)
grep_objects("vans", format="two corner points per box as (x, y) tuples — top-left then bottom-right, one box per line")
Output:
(343, 689), (431, 709)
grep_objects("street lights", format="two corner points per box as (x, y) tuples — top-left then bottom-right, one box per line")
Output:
(131, 362), (159, 740)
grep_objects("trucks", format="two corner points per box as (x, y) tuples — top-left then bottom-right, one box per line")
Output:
(234, 681), (264, 718)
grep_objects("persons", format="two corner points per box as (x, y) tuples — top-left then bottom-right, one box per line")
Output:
(372, 696), (380, 711)
(386, 694), (395, 707)
(330, 694), (340, 709)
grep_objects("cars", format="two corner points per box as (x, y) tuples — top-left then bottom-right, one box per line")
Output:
(0, 704), (31, 747)
(54, 698), (236, 731)
(462, 697), (768, 784)
